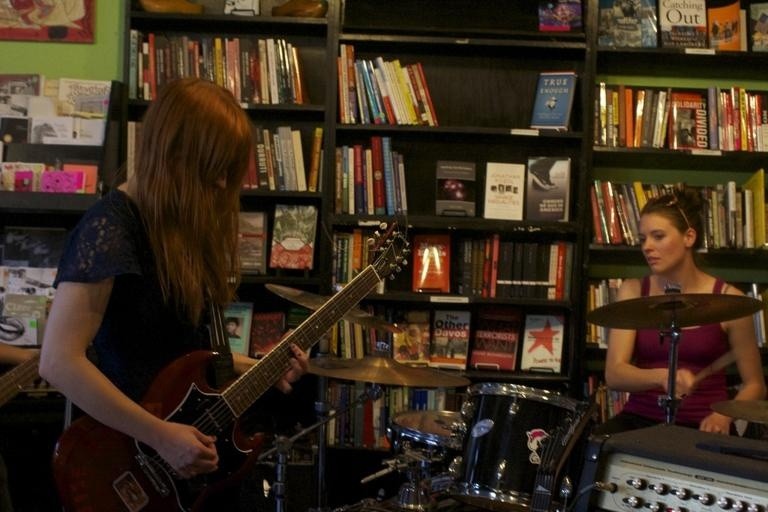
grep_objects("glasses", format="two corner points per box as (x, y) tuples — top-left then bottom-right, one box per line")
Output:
(659, 194), (691, 229)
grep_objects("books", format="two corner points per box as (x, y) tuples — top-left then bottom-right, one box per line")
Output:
(0, 2), (330, 359)
(320, 46), (767, 446)
(538, 2), (767, 53)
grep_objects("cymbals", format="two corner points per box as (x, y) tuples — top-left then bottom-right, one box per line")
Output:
(307, 357), (472, 388)
(587, 294), (763, 330)
(268, 284), (403, 335)
(710, 401), (766, 423)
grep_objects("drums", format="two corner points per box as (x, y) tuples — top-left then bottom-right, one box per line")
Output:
(386, 411), (468, 510)
(451, 382), (588, 508)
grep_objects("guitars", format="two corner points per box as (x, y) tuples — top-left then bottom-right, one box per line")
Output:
(52, 208), (410, 512)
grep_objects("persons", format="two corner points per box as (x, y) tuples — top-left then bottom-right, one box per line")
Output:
(37, 75), (312, 510)
